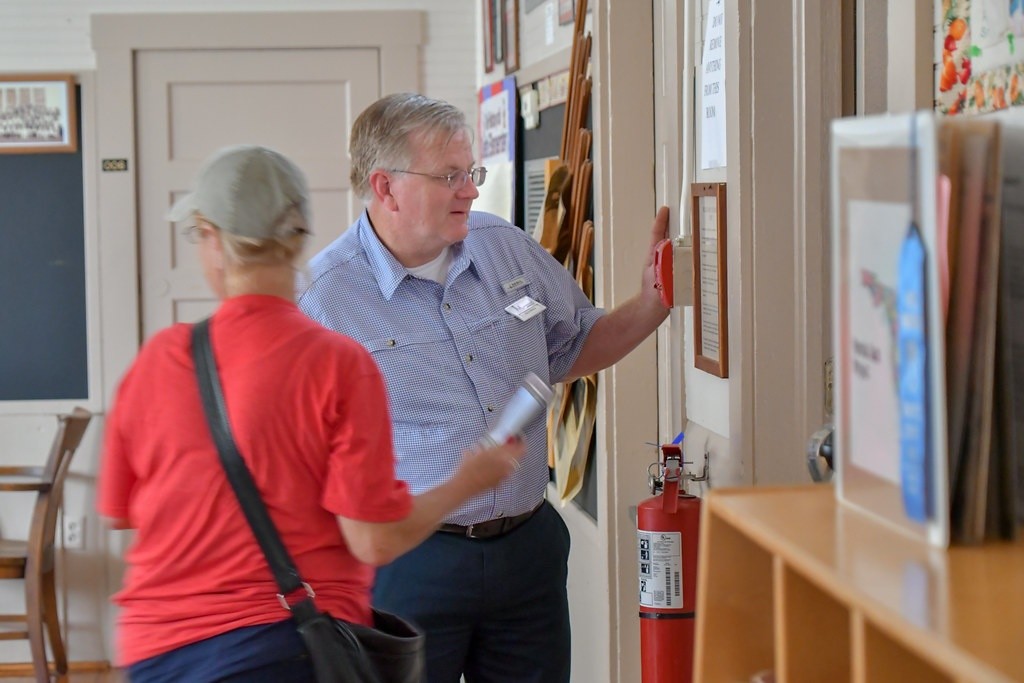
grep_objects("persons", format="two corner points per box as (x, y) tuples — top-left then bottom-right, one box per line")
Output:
(95, 144), (522, 683)
(297, 93), (670, 683)
(0, 107), (62, 141)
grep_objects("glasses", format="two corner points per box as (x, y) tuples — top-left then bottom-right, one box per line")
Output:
(390, 167), (489, 191)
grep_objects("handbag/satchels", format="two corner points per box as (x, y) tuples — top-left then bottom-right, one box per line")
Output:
(289, 598), (427, 683)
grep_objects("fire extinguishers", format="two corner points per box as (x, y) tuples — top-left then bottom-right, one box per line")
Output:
(638, 445), (703, 683)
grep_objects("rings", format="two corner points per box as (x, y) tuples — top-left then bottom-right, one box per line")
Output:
(510, 458), (519, 466)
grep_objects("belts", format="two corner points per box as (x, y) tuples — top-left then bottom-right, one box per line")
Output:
(440, 522), (521, 539)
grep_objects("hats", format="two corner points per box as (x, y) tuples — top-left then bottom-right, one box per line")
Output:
(163, 144), (313, 248)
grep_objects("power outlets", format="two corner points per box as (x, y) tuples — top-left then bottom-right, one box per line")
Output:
(55, 517), (87, 550)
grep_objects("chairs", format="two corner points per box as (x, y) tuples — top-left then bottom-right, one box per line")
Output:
(0, 407), (92, 683)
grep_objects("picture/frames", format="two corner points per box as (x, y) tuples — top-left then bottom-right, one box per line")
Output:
(692, 182), (725, 378)
(0, 73), (78, 154)
(480, 0), (520, 76)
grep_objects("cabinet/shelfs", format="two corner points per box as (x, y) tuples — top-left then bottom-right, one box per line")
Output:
(693, 482), (1024, 683)
